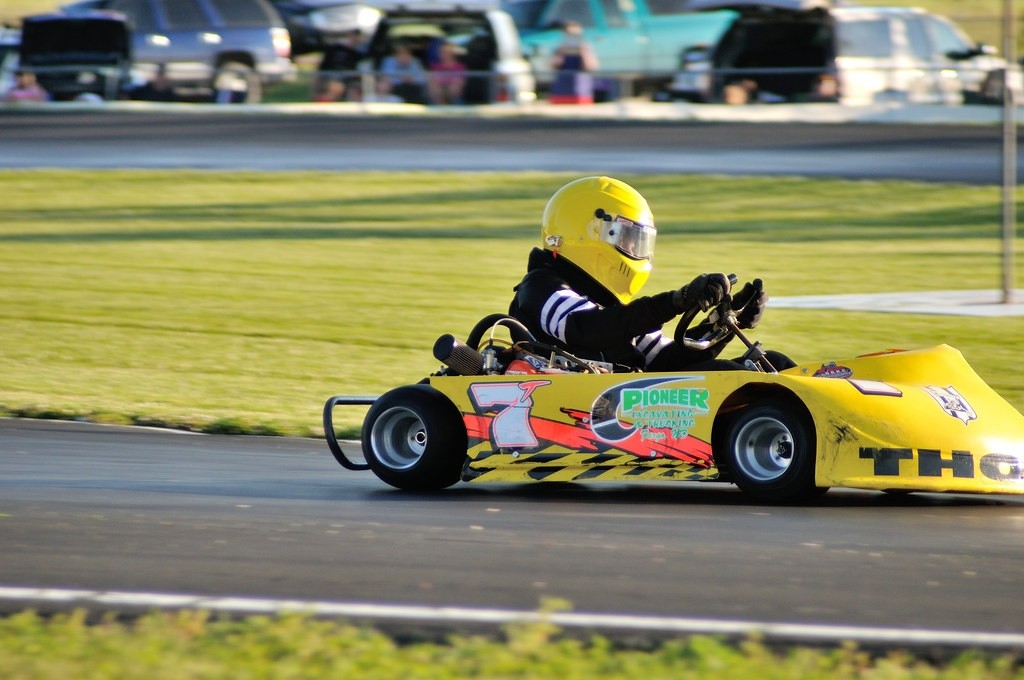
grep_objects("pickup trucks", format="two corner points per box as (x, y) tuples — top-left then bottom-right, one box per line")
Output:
(446, 0), (740, 99)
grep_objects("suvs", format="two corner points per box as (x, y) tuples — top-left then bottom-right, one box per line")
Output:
(19, 0), (293, 104)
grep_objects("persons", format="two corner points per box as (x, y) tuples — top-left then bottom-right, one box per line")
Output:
(5, 65), (54, 102)
(554, 21), (599, 104)
(509, 175), (795, 372)
(317, 28), (517, 106)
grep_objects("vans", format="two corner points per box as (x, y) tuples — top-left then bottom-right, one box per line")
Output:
(670, 6), (1023, 109)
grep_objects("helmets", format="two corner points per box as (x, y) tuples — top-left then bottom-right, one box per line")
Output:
(542, 176), (657, 304)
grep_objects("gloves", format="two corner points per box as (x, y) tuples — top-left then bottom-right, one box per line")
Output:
(674, 273), (730, 314)
(731, 282), (769, 330)
